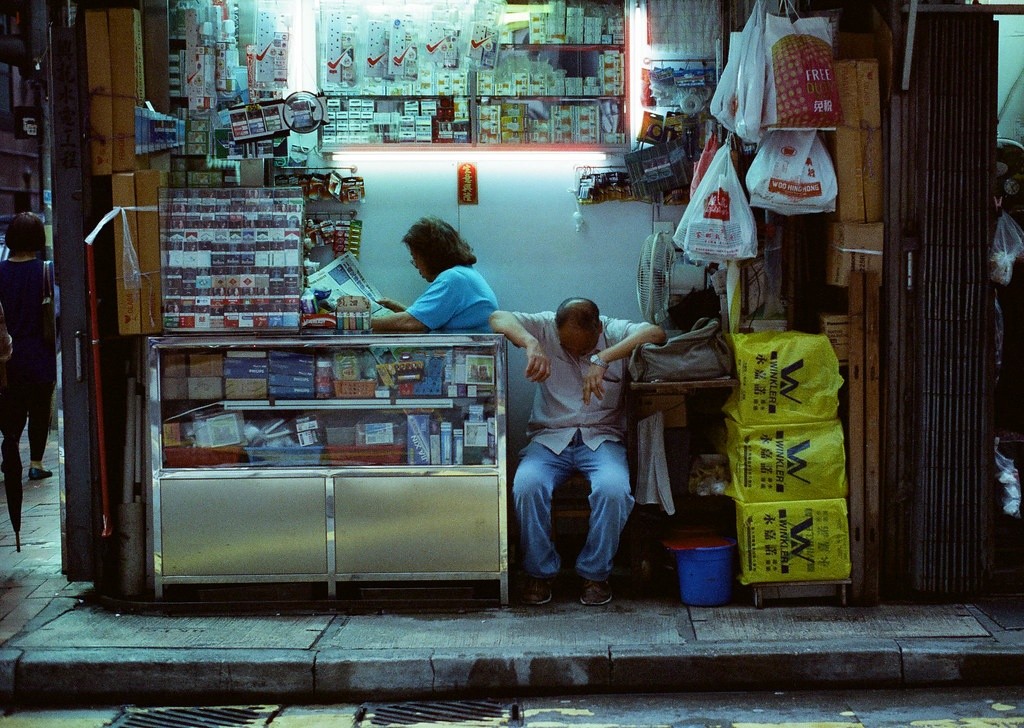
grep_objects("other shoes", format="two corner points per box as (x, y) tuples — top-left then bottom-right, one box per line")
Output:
(28, 467), (53, 481)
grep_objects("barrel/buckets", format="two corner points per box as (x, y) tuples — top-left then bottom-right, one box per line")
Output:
(676, 537), (737, 607)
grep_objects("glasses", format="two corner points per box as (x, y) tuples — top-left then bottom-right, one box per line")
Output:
(410, 256), (421, 269)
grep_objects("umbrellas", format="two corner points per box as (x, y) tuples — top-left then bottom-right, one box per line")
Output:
(0, 358), (34, 550)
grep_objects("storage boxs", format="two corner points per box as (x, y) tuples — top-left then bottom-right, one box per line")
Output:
(642, 393), (687, 428)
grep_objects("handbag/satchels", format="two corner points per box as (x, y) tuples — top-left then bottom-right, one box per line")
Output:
(690, 132), (738, 203)
(41, 260), (57, 348)
(989, 210), (1023, 288)
(671, 142), (761, 261)
(708, 0), (770, 145)
(745, 131), (838, 216)
(763, 0), (846, 134)
(992, 437), (1022, 522)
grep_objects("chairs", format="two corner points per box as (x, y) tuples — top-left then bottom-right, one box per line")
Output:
(552, 473), (594, 519)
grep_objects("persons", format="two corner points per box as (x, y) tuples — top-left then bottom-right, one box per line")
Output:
(371, 216), (500, 333)
(489, 298), (667, 604)
(0, 213), (60, 482)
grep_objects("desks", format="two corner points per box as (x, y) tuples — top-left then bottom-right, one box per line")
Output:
(627, 375), (735, 393)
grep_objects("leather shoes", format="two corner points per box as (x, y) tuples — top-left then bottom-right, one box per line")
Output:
(520, 574), (613, 605)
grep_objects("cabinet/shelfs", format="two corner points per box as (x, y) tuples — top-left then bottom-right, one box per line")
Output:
(148, 329), (513, 606)
(241, 1), (631, 151)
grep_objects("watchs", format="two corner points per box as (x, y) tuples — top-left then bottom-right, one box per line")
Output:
(589, 354), (609, 369)
